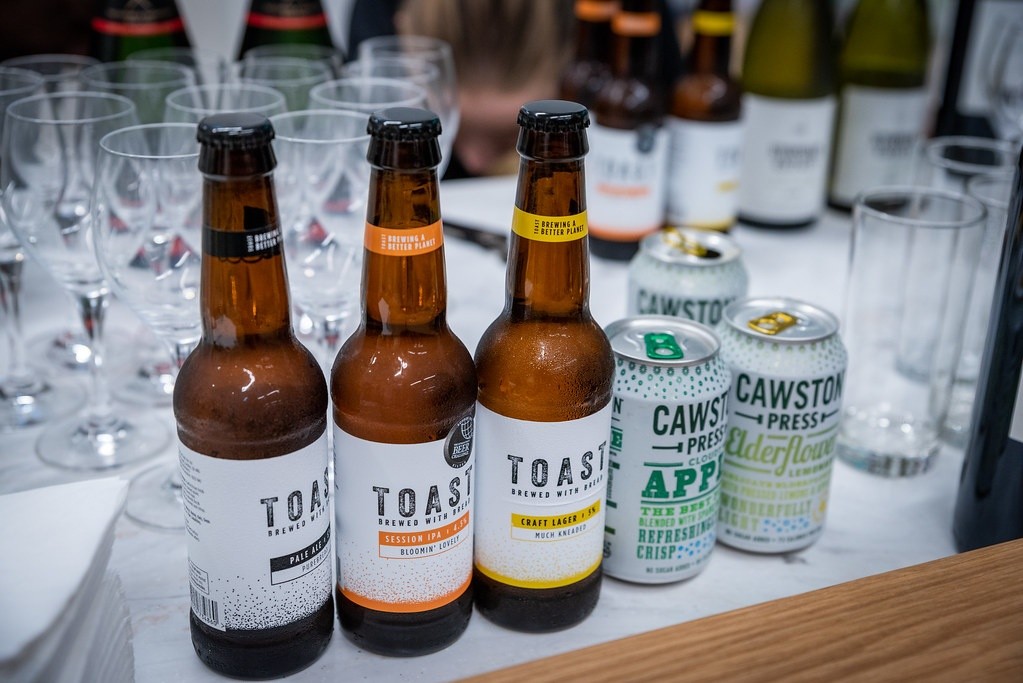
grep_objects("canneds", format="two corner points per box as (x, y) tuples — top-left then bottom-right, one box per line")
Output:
(600, 313), (733, 581)
(714, 293), (850, 553)
(625, 224), (749, 326)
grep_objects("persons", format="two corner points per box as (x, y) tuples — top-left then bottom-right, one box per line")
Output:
(346, 0), (696, 183)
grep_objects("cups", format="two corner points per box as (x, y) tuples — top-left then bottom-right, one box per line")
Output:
(841, 186), (987, 480)
(936, 174), (1014, 447)
(892, 132), (1016, 383)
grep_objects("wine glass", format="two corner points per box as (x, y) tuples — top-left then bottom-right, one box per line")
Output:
(0, 33), (464, 531)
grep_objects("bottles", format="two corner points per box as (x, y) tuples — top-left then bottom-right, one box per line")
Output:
(558, 0), (931, 263)
(329, 105), (475, 659)
(171, 107), (340, 681)
(474, 97), (618, 633)
(952, 144), (1023, 555)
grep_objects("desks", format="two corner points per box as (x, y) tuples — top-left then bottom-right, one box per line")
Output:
(446, 537), (1023, 683)
